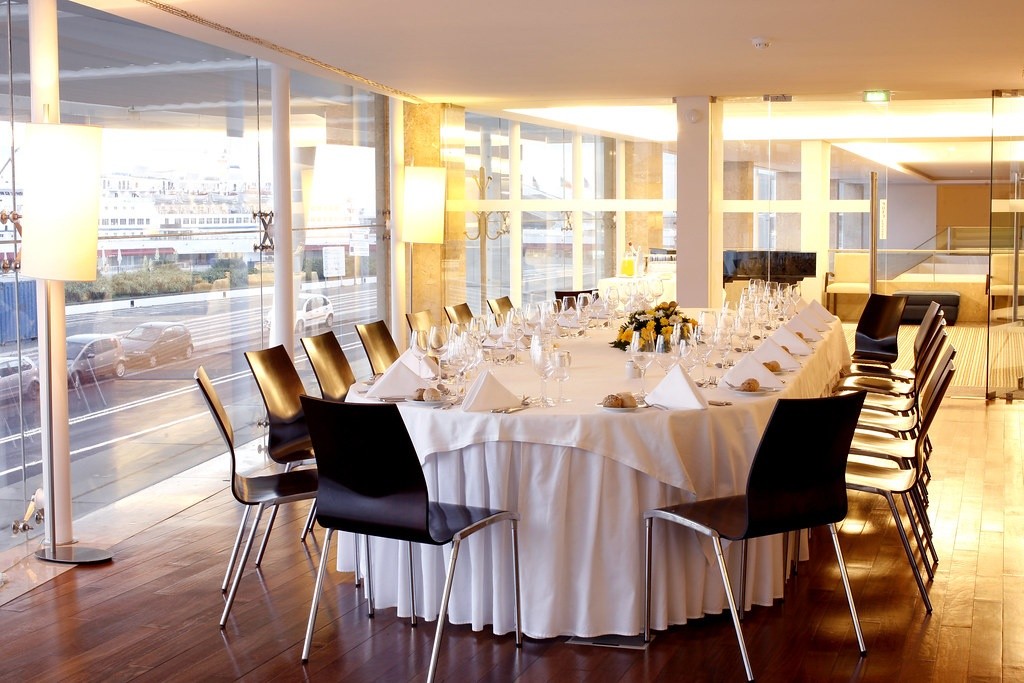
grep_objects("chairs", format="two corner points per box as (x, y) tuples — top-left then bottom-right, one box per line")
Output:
(784, 291), (958, 615)
(988, 254), (1023, 320)
(241, 343), (323, 568)
(825, 253), (869, 316)
(293, 286), (599, 403)
(300, 393), (522, 683)
(191, 362), (321, 632)
(648, 389), (868, 682)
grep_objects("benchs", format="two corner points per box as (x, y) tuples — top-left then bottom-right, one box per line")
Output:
(892, 293), (960, 326)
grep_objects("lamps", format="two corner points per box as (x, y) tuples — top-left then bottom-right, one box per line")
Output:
(863, 90), (892, 101)
(25, 120), (114, 567)
(400, 164), (446, 340)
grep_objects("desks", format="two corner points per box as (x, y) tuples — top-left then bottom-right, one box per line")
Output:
(591, 268), (677, 302)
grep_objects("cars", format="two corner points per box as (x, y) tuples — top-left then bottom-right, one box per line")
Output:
(264, 294), (333, 333)
(1, 356), (39, 405)
(66, 333), (124, 386)
(119, 322), (194, 368)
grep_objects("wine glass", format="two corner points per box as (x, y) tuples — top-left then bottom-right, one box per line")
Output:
(410, 277), (802, 411)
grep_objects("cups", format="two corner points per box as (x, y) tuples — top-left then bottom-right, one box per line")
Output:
(621, 250), (634, 275)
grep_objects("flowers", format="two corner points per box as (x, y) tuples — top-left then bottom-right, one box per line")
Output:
(608, 299), (700, 353)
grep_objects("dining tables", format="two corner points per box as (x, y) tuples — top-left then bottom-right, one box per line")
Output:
(330, 307), (848, 638)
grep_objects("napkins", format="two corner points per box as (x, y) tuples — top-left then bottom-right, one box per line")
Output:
(712, 298), (834, 390)
(460, 370), (525, 413)
(364, 361), (432, 398)
(642, 362), (706, 410)
(382, 347), (444, 381)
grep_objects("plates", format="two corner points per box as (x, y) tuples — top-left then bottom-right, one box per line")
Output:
(729, 387), (768, 394)
(595, 403), (638, 410)
(404, 397), (456, 405)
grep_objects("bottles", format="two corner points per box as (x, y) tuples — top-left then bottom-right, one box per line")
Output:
(628, 241), (638, 254)
(626, 360), (641, 378)
